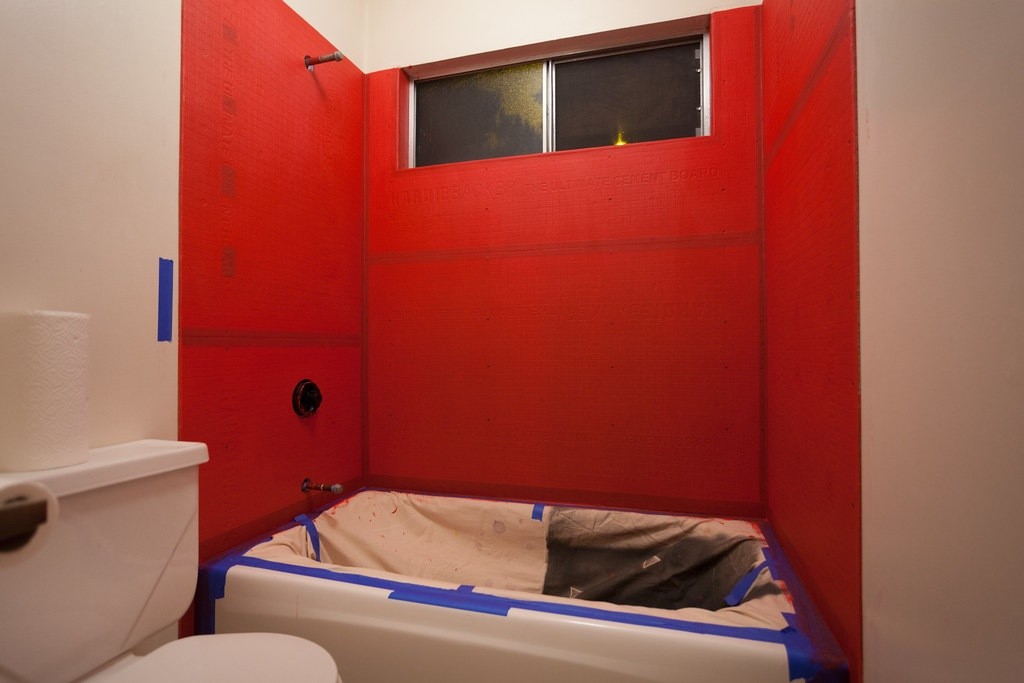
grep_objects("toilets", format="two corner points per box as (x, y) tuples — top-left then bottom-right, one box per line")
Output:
(0, 437), (344, 683)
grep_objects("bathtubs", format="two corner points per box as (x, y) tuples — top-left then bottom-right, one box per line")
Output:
(195, 486), (851, 683)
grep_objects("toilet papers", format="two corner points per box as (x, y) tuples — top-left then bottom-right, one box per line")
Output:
(1, 309), (92, 475)
